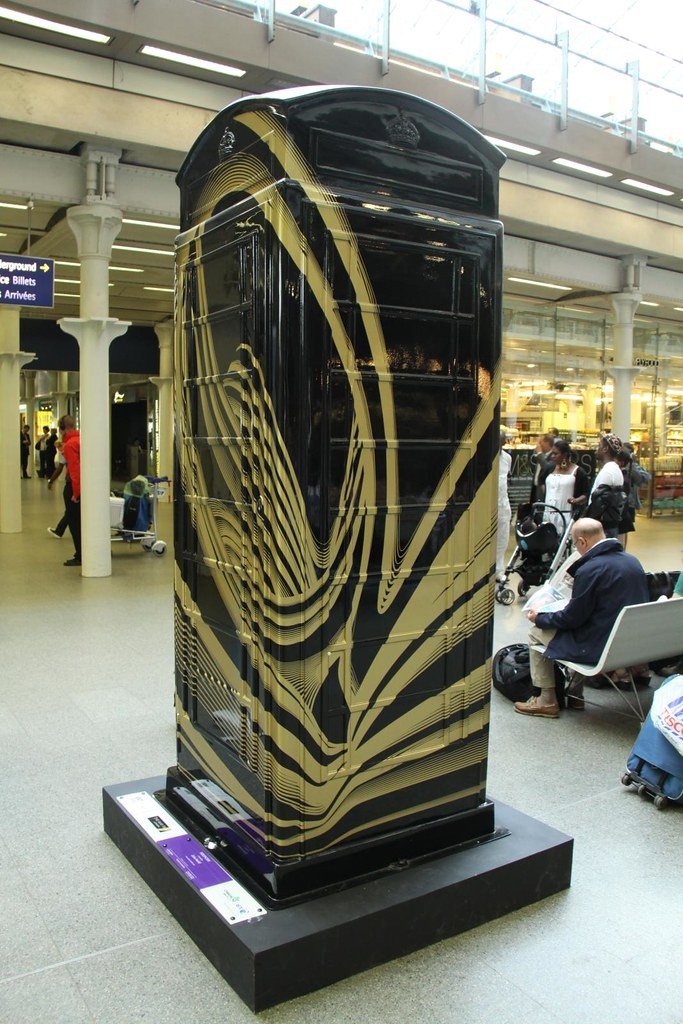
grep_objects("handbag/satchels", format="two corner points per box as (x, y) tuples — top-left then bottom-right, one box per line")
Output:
(36, 441), (41, 451)
(645, 571), (680, 602)
(492, 644), (566, 712)
(110, 492), (125, 529)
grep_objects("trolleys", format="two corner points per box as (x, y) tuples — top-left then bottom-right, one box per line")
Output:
(111, 476), (171, 557)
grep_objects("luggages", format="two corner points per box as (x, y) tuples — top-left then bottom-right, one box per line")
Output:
(621, 675), (683, 809)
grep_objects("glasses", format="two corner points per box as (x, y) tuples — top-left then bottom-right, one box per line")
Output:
(572, 540), (578, 551)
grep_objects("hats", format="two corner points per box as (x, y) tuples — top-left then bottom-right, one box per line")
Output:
(600, 432), (621, 455)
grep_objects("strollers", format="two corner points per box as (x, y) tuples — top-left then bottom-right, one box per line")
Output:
(496, 499), (583, 605)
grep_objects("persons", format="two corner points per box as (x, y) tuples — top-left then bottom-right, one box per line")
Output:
(615, 450), (636, 551)
(586, 433), (626, 541)
(622, 442), (651, 522)
(36, 426), (58, 480)
(549, 428), (562, 443)
(495, 430), (512, 582)
(530, 436), (555, 503)
(520, 519), (537, 533)
(541, 441), (588, 537)
(20, 425), (32, 478)
(512, 518), (650, 719)
(607, 571), (683, 690)
(47, 416), (81, 566)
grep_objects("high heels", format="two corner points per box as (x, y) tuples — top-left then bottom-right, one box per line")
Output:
(597, 671), (631, 691)
(633, 670), (651, 685)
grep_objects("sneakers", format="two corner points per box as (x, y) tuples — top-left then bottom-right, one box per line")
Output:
(565, 690), (584, 710)
(513, 696), (560, 717)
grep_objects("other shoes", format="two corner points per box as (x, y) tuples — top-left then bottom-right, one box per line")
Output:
(47, 527), (62, 538)
(64, 559), (81, 566)
(496, 574), (509, 583)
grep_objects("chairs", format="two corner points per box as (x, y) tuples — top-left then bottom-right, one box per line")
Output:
(531, 597), (683, 730)
